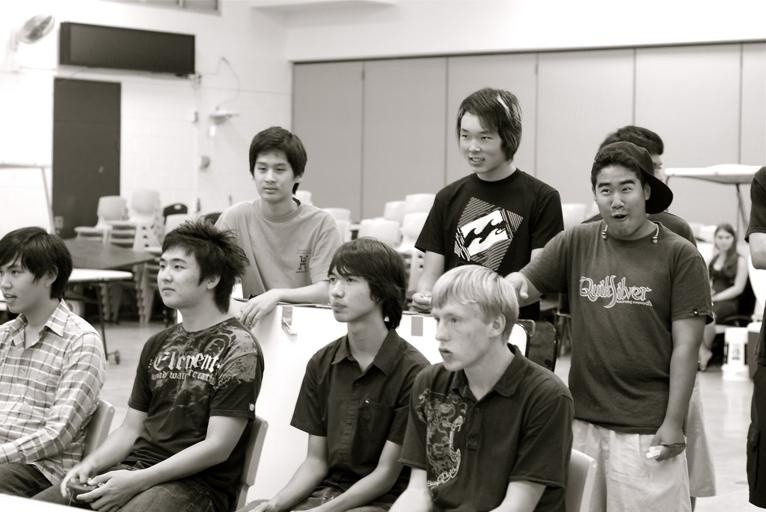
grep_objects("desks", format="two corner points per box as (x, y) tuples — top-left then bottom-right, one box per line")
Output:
(71, 267), (132, 364)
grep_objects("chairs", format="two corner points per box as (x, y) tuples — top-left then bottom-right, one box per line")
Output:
(72, 189), (166, 324)
(298, 191), (437, 289)
(78, 399), (117, 463)
(233, 416), (269, 511)
(566, 449), (600, 510)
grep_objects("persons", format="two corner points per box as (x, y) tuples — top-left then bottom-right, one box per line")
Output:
(211, 126), (343, 330)
(249, 237), (432, 512)
(501, 141), (713, 511)
(0, 225), (109, 503)
(411, 87), (567, 367)
(387, 265), (573, 512)
(30, 217), (264, 512)
(699, 220), (749, 371)
(744, 158), (765, 509)
(578, 124), (718, 512)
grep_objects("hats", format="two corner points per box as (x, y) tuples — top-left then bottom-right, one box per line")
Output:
(601, 141), (673, 214)
